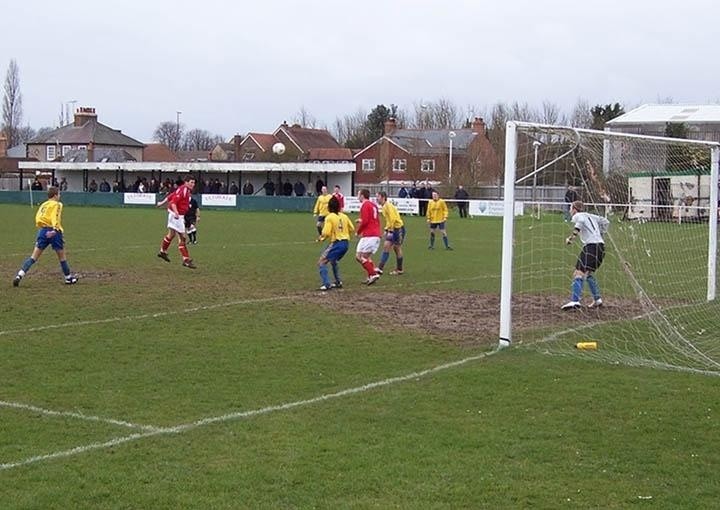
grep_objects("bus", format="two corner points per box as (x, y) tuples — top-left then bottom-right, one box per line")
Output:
(374, 180), (442, 197)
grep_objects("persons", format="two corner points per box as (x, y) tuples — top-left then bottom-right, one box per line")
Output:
(13, 187), (78, 286)
(397, 183), (435, 216)
(31, 176), (324, 196)
(564, 186), (577, 223)
(454, 185), (469, 218)
(374, 192), (405, 275)
(355, 189), (381, 285)
(332, 186), (344, 212)
(157, 176), (197, 269)
(313, 186), (332, 240)
(316, 196), (354, 291)
(426, 190), (450, 250)
(184, 192), (200, 244)
(560, 200), (610, 310)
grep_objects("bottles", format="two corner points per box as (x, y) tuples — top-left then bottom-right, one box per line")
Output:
(575, 341), (597, 349)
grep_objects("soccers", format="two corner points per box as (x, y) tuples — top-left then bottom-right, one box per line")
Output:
(273, 143), (286, 155)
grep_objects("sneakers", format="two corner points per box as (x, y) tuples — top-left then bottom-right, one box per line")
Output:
(320, 281), (343, 290)
(183, 258), (196, 268)
(362, 267), (383, 285)
(65, 275), (78, 284)
(561, 299), (603, 310)
(14, 275), (21, 286)
(389, 269), (404, 275)
(158, 251), (170, 262)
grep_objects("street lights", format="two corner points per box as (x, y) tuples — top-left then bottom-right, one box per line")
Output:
(420, 103), (429, 130)
(174, 109), (183, 151)
(530, 138), (543, 219)
(448, 129), (458, 185)
